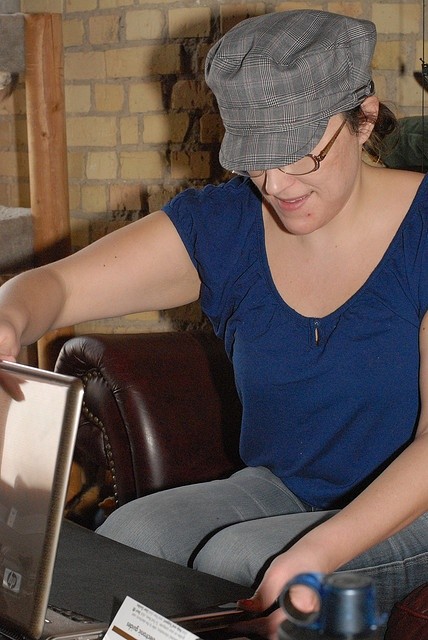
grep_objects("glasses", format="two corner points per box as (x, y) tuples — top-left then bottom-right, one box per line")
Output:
(226, 113), (349, 179)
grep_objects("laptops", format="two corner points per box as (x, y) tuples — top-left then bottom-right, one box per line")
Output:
(1, 360), (279, 640)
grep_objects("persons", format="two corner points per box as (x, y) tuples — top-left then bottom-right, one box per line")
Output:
(0, 8), (428, 640)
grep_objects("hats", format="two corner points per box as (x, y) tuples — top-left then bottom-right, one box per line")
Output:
(203, 9), (376, 171)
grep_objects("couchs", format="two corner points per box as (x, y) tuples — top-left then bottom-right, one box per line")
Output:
(48, 334), (428, 638)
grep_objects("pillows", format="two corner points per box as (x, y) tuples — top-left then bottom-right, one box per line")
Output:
(365, 116), (427, 168)
